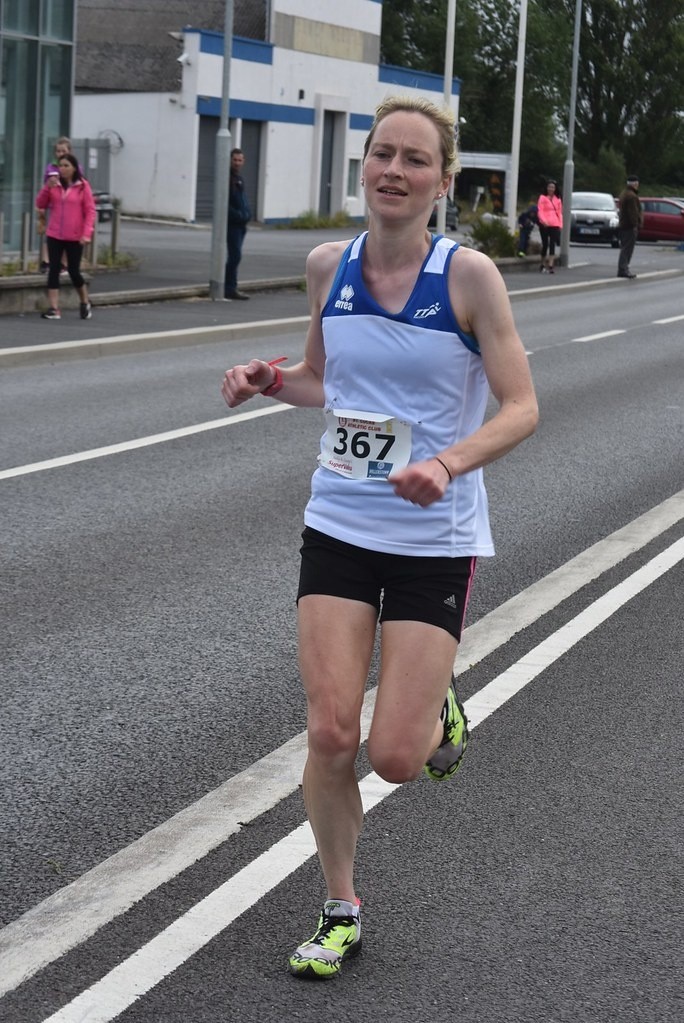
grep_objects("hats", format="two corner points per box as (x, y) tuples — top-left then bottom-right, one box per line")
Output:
(627, 174), (638, 182)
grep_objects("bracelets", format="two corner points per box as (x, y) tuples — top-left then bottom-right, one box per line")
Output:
(434, 456), (452, 481)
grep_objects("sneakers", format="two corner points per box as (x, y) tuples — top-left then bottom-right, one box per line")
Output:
(422, 671), (468, 780)
(42, 309), (61, 320)
(290, 898), (362, 979)
(80, 298), (92, 321)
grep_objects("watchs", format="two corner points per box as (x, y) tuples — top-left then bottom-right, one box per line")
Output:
(259, 356), (288, 396)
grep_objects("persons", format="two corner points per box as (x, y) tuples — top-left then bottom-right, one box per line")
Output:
(518, 180), (563, 274)
(616, 175), (643, 279)
(221, 93), (539, 981)
(224, 148), (252, 300)
(35, 137), (97, 319)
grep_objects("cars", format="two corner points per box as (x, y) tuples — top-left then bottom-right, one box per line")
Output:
(570, 192), (620, 248)
(427, 195), (460, 231)
(614, 197), (684, 242)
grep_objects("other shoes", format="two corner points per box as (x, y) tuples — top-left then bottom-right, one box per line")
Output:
(548, 266), (554, 273)
(225, 289), (249, 300)
(518, 251), (526, 257)
(39, 261), (50, 275)
(539, 264), (546, 273)
(619, 268), (636, 278)
